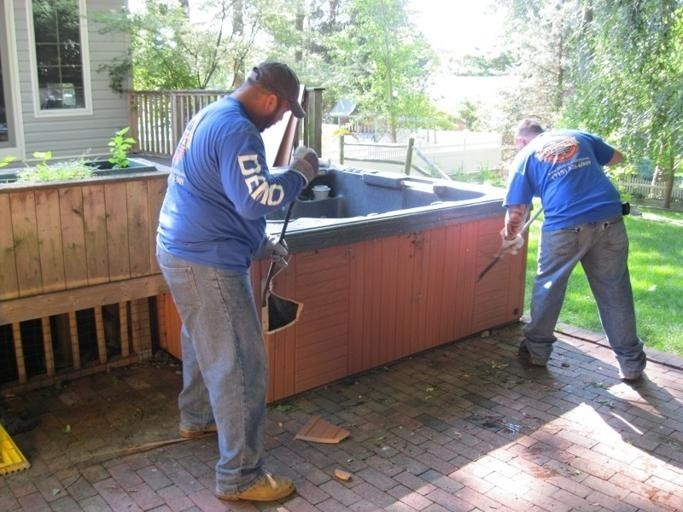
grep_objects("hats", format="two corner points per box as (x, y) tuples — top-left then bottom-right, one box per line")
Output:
(252, 61), (306, 118)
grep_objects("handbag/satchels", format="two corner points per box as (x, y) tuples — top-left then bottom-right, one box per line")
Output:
(621, 202), (630, 214)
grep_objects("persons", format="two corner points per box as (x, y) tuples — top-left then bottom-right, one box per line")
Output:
(154, 60), (321, 503)
(496, 117), (651, 384)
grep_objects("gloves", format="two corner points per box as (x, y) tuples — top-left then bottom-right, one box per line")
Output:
(502, 232), (523, 254)
(290, 145), (319, 185)
(261, 234), (288, 266)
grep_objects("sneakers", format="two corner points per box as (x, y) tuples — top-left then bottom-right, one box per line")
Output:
(619, 370), (641, 380)
(519, 338), (553, 367)
(215, 473), (296, 502)
(179, 420), (216, 437)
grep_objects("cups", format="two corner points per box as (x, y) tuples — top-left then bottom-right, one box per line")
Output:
(312, 185), (331, 199)
